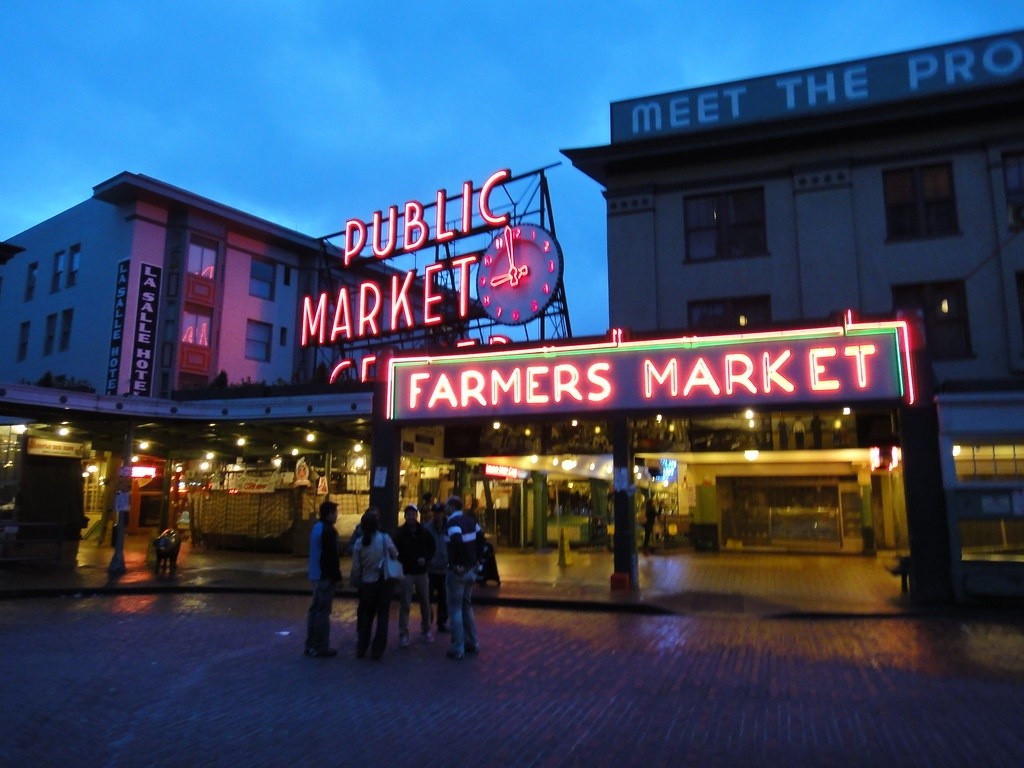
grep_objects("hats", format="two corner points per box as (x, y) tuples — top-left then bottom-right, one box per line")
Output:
(422, 492), (433, 500)
(431, 504), (444, 511)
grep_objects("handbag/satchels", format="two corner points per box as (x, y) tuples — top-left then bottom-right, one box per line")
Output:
(378, 533), (403, 580)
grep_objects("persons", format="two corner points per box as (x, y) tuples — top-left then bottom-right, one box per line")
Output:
(349, 512), (400, 659)
(446, 495), (489, 660)
(347, 506), (380, 556)
(424, 503), (450, 634)
(420, 493), (435, 523)
(392, 504), (436, 648)
(643, 500), (655, 548)
(303, 502), (344, 657)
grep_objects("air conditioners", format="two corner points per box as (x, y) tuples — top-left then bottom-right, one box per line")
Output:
(1007, 204), (1024, 230)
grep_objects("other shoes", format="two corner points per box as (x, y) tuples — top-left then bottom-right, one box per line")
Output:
(371, 648), (381, 662)
(446, 647), (463, 659)
(400, 633), (409, 647)
(357, 647), (367, 657)
(464, 642), (478, 652)
(423, 629), (435, 643)
(437, 625), (450, 634)
(303, 648), (336, 658)
